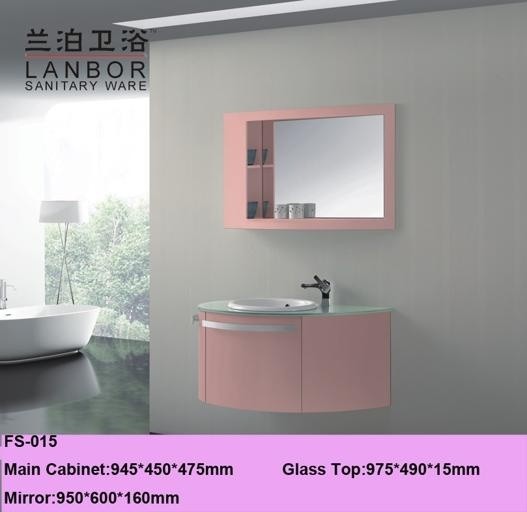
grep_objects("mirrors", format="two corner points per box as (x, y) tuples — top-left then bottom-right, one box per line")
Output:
(220, 101), (399, 232)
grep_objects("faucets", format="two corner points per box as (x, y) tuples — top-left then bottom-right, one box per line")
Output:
(300, 275), (331, 299)
(0, 414), (14, 426)
(0, 279), (16, 309)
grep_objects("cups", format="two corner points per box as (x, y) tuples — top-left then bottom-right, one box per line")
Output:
(274, 203), (305, 220)
(248, 201), (258, 219)
(304, 202), (316, 218)
(247, 148), (257, 165)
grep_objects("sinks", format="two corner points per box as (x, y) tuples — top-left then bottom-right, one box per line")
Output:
(226, 299), (319, 312)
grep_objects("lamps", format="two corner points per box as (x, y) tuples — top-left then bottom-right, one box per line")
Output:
(38, 197), (82, 305)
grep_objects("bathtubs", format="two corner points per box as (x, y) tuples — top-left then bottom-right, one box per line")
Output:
(0, 303), (101, 366)
(0, 352), (100, 414)
